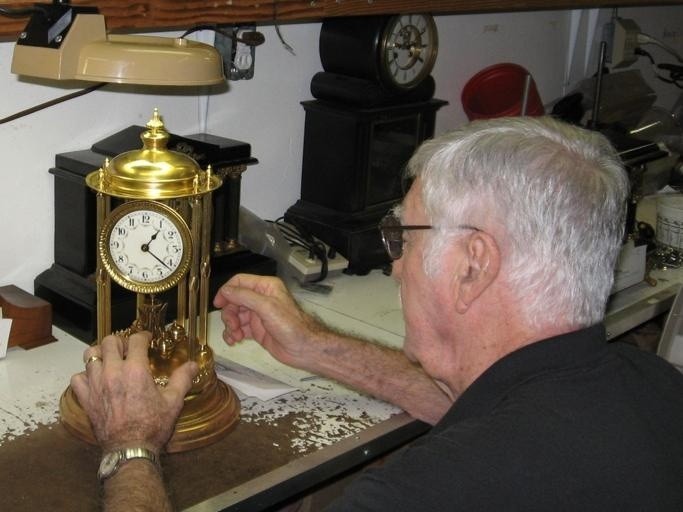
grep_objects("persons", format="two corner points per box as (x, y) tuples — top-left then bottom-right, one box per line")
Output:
(70, 114), (683, 512)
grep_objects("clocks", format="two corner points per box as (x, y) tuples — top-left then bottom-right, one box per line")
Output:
(284, 15), (450, 275)
(61, 108), (242, 457)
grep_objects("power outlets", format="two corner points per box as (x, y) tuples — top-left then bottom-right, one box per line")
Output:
(474, 21), (504, 39)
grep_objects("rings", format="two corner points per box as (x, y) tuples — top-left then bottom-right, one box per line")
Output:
(83, 355), (103, 369)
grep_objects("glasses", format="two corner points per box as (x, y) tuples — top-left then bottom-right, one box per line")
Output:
(380, 215), (486, 260)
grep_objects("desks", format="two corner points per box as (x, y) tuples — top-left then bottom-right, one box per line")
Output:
(3, 196), (682, 508)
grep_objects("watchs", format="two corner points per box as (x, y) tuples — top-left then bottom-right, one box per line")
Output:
(89, 447), (165, 484)
